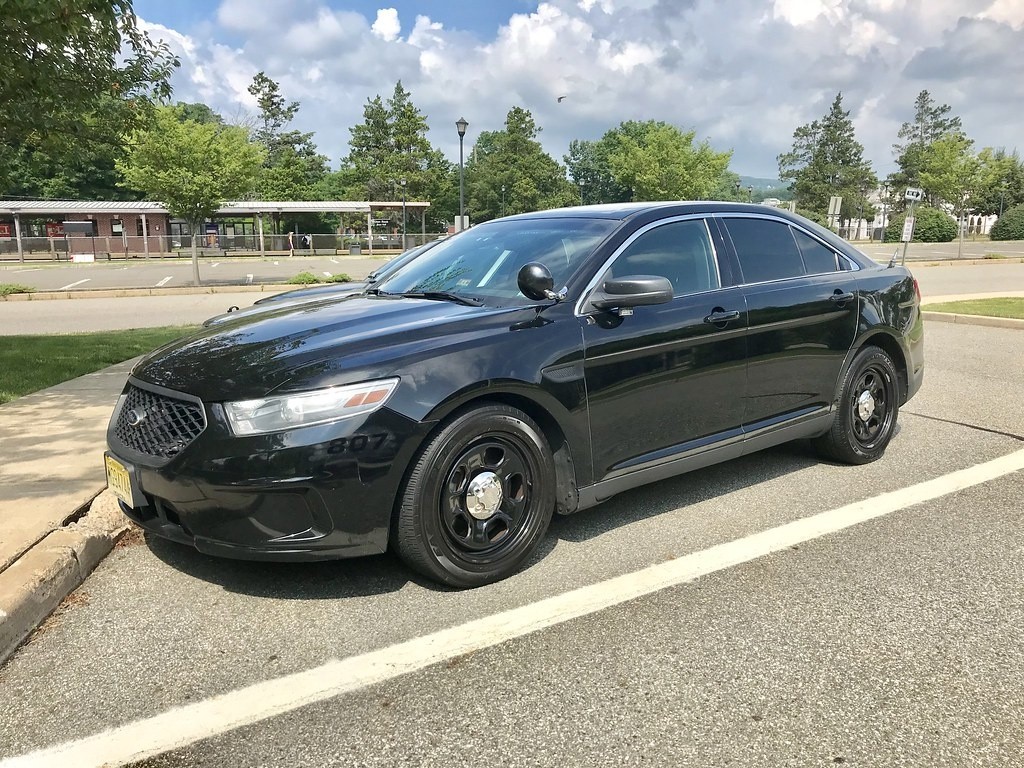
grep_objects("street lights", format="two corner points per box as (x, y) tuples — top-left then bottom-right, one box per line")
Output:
(455, 116), (469, 232)
(501, 185), (506, 216)
(400, 178), (409, 252)
(999, 176), (1008, 222)
(882, 179), (890, 243)
(735, 178), (742, 203)
(748, 185), (753, 203)
(858, 186), (865, 240)
(579, 178), (585, 206)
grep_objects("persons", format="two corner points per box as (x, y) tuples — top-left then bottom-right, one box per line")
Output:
(302, 233), (312, 249)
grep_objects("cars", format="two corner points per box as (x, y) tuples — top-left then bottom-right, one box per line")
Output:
(102, 197), (927, 595)
(341, 233), (395, 241)
(171, 240), (181, 248)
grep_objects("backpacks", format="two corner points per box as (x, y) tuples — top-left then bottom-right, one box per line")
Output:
(302, 236), (307, 247)
